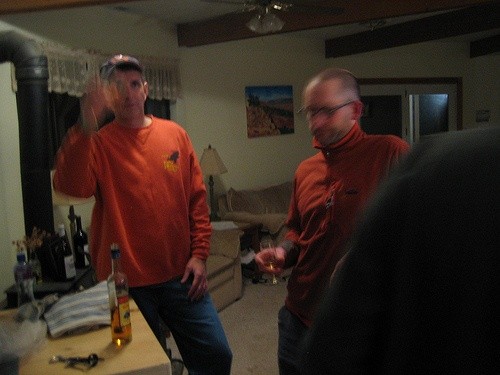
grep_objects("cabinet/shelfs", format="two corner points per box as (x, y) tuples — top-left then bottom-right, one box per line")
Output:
(6, 252), (98, 309)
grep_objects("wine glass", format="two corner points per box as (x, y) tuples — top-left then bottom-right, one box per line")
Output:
(260, 240), (281, 288)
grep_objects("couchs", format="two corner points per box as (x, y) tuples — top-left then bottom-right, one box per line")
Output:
(218, 182), (299, 269)
(206, 228), (243, 312)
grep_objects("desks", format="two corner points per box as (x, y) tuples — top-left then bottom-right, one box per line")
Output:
(1, 290), (173, 375)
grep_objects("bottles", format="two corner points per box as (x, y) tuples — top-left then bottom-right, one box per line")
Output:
(14, 253), (34, 307)
(57, 224), (76, 281)
(107, 249), (132, 345)
(74, 217), (89, 269)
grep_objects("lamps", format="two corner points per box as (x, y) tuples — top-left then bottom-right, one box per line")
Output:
(237, 0), (292, 34)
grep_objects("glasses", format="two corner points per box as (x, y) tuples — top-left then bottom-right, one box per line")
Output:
(297, 100), (354, 120)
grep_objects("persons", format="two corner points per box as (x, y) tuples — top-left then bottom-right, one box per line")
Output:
(254, 66), (411, 375)
(299, 125), (499, 375)
(52, 54), (233, 375)
